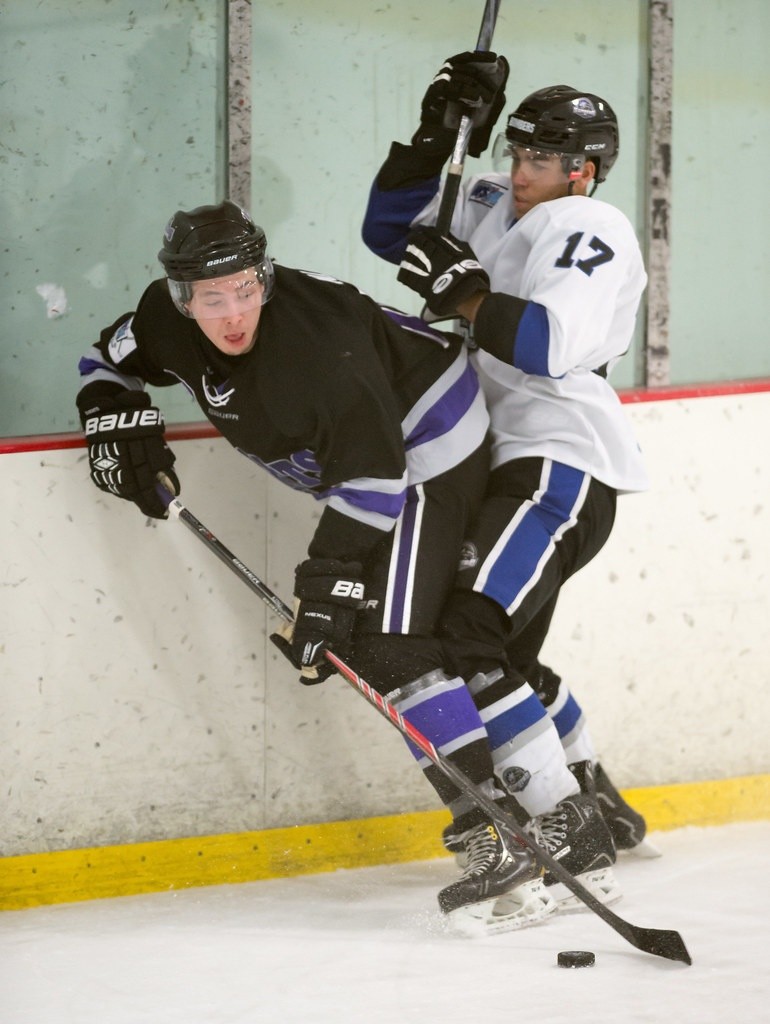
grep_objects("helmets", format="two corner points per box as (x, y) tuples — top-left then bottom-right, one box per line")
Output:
(506, 84), (619, 183)
(157, 200), (267, 302)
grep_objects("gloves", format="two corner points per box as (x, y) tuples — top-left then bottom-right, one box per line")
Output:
(412, 50), (508, 159)
(397, 224), (490, 314)
(270, 559), (376, 691)
(76, 380), (181, 520)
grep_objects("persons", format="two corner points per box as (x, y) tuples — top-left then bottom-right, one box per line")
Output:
(360, 49), (660, 916)
(70, 200), (558, 937)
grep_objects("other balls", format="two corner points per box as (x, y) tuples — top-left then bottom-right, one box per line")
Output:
(558, 951), (596, 969)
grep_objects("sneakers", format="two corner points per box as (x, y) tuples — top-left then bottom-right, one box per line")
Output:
(525, 799), (623, 917)
(437, 811), (554, 942)
(569, 763), (663, 856)
(442, 825), (468, 867)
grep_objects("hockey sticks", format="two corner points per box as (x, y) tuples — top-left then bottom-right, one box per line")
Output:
(435, 0), (501, 237)
(157, 479), (694, 966)
